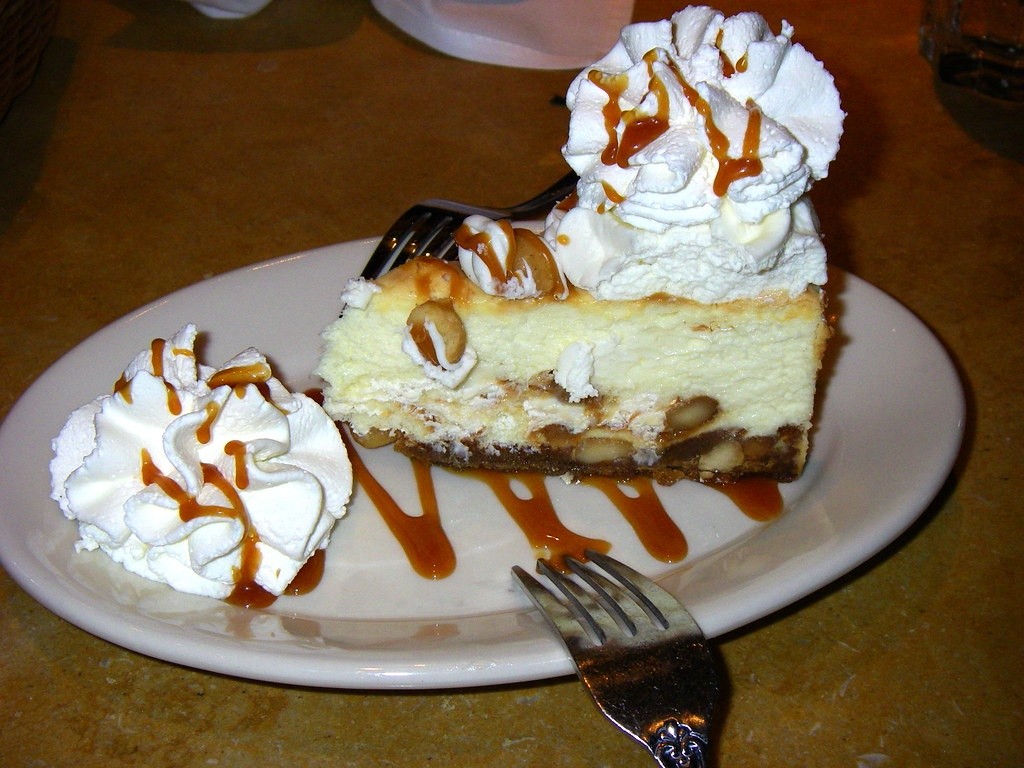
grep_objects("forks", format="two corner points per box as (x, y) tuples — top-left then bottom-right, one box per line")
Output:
(339, 169), (580, 317)
(512, 549), (732, 768)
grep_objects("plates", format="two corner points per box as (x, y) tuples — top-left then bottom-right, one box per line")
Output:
(0, 220), (963, 689)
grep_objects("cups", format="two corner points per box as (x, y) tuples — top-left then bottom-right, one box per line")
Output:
(919, 0), (1024, 102)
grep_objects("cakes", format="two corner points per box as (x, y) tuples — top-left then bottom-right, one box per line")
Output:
(312, 6), (847, 481)
